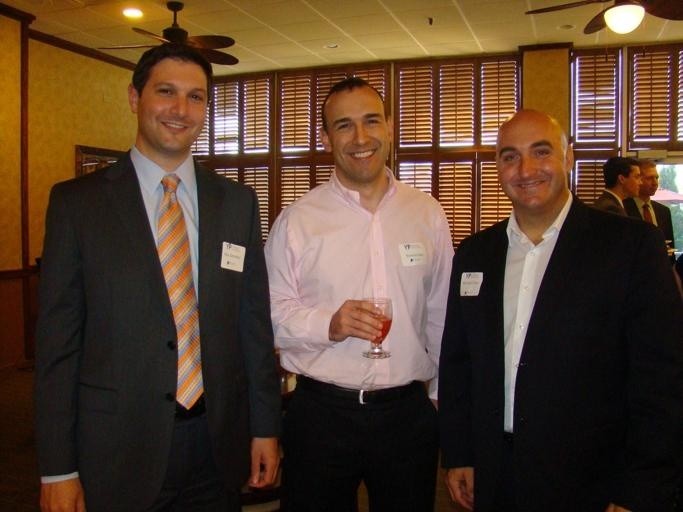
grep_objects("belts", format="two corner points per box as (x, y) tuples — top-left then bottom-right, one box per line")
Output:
(308, 379), (422, 405)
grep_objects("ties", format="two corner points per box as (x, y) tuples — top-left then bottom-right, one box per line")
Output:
(641, 200), (653, 226)
(157, 172), (204, 410)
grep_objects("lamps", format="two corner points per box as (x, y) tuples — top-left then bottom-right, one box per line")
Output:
(602, 3), (643, 37)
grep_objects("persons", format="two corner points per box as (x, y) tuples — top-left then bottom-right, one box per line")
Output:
(623, 159), (675, 267)
(262, 76), (456, 512)
(31, 43), (284, 512)
(670, 253), (683, 300)
(592, 156), (643, 218)
(437, 107), (683, 512)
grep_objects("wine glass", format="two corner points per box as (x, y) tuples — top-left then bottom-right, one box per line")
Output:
(362, 297), (391, 359)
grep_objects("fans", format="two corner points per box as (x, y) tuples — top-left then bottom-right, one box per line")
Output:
(525, 0), (682, 39)
(95, 2), (237, 70)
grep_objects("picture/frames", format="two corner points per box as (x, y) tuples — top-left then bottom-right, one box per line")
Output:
(73, 143), (127, 180)
